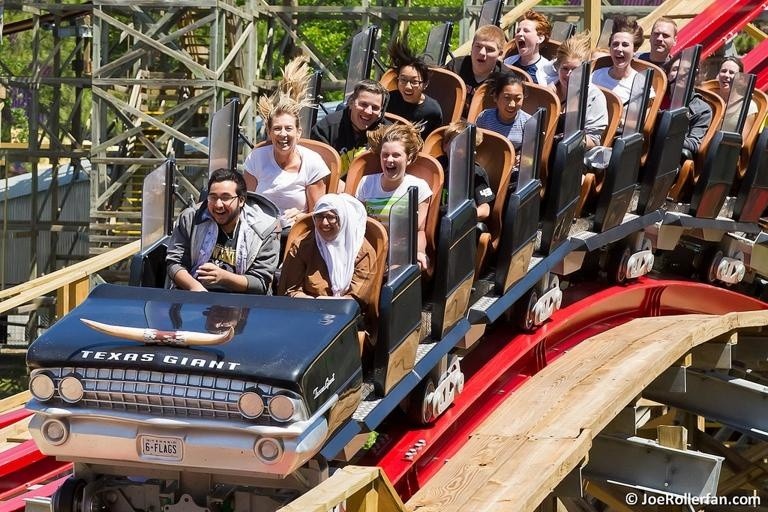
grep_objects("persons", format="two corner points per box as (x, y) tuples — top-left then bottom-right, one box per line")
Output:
(165, 6), (767, 335)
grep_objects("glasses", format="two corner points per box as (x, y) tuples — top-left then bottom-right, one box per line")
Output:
(314, 214), (339, 224)
(207, 195), (239, 202)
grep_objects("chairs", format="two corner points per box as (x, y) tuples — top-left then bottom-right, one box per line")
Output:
(198, 38), (768, 348)
